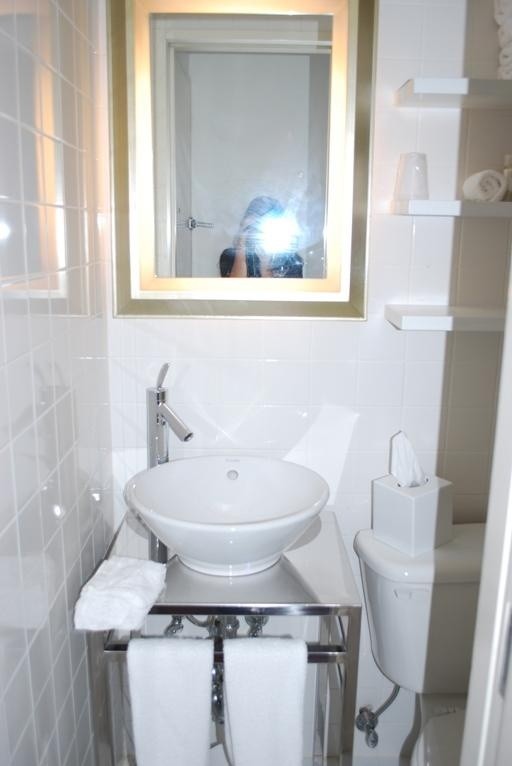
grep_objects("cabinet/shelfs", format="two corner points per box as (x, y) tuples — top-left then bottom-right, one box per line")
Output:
(381, 67), (511, 343)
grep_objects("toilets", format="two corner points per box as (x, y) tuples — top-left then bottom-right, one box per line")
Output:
(352, 520), (487, 766)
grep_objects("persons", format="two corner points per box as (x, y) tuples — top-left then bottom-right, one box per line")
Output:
(218, 195), (304, 278)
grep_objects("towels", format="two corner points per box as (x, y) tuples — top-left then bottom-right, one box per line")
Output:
(123, 629), (308, 766)
(456, 168), (509, 207)
(490, 0), (511, 82)
(73, 555), (173, 642)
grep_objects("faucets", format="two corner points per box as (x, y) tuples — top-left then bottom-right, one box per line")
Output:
(158, 402), (195, 445)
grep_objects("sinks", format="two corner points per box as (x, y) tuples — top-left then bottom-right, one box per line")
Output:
(125, 455), (331, 576)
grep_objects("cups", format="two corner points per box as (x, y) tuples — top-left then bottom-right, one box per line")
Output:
(395, 152), (432, 201)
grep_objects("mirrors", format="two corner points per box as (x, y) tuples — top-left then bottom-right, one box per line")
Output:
(105, 0), (377, 330)
